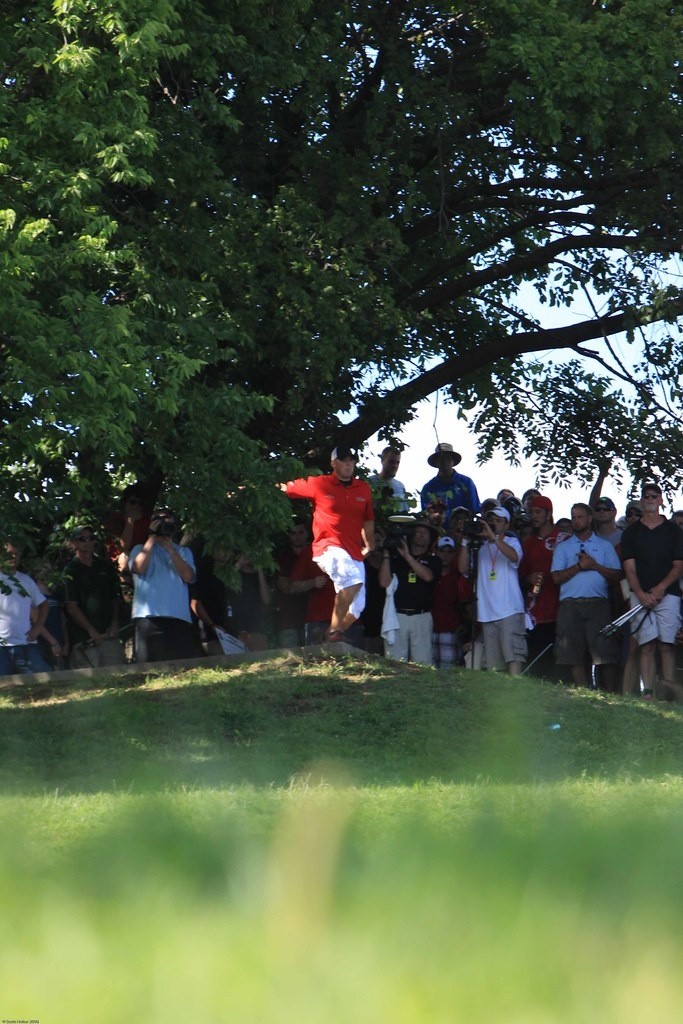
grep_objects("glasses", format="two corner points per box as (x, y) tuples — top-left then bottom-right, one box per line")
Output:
(75, 535), (93, 542)
(595, 506), (613, 512)
(644, 493), (658, 499)
(579, 544), (585, 559)
(625, 512), (642, 517)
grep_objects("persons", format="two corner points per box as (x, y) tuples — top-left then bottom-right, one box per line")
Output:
(551, 503), (621, 691)
(620, 484), (683, 702)
(655, 510), (683, 700)
(361, 444), (573, 681)
(0, 509), (335, 675)
(227, 445), (375, 642)
(594, 497), (644, 695)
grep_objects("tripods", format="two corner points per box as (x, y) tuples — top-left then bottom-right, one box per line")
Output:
(600, 604), (643, 639)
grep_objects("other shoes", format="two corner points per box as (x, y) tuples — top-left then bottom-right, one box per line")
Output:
(325, 628), (343, 642)
(641, 693), (653, 703)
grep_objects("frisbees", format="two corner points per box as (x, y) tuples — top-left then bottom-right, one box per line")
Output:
(387, 515), (417, 523)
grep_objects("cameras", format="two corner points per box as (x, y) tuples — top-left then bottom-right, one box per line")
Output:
(153, 517), (176, 536)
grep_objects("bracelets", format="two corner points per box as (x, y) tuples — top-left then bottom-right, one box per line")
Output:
(578, 562), (583, 570)
(383, 556), (390, 559)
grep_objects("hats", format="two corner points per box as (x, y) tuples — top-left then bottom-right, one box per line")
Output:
(438, 537), (455, 548)
(485, 506), (511, 523)
(501, 496), (522, 508)
(450, 506), (470, 520)
(428, 444), (462, 468)
(408, 515), (438, 532)
(481, 498), (501, 511)
(428, 498), (447, 509)
(330, 445), (359, 461)
(626, 500), (644, 512)
(596, 497), (615, 509)
(70, 524), (95, 540)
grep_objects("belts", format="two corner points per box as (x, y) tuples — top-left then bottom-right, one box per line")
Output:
(563, 597), (601, 603)
(396, 608), (429, 616)
